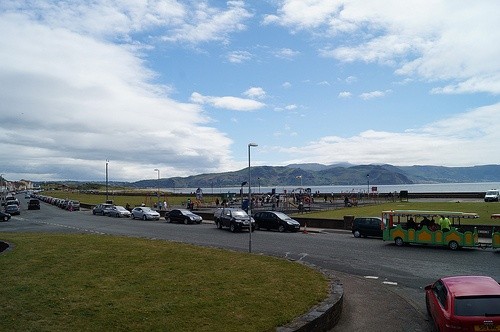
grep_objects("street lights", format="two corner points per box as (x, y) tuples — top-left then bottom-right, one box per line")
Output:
(247, 142), (259, 253)
(154, 169), (160, 212)
(366, 174), (370, 199)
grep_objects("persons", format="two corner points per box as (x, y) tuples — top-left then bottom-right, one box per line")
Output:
(404, 217), (419, 231)
(187, 198), (191, 206)
(437, 215), (452, 233)
(419, 216), (435, 231)
(296, 201), (305, 215)
(159, 201), (167, 211)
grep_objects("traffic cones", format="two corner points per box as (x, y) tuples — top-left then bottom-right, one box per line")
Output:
(302, 224), (309, 235)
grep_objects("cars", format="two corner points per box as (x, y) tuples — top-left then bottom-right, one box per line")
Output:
(107, 206), (130, 218)
(164, 209), (203, 225)
(253, 210), (301, 233)
(6, 205), (21, 215)
(92, 203), (115, 216)
(484, 190), (500, 202)
(0, 212), (11, 222)
(424, 276), (500, 332)
(130, 207), (160, 221)
(0, 189), (80, 212)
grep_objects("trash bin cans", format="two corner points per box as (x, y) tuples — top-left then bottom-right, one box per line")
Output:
(344, 215), (354, 230)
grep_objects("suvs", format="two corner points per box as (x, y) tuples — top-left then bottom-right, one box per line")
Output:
(27, 199), (41, 210)
(214, 208), (256, 232)
(351, 217), (397, 238)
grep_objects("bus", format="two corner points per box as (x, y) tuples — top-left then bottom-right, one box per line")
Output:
(381, 209), (500, 251)
